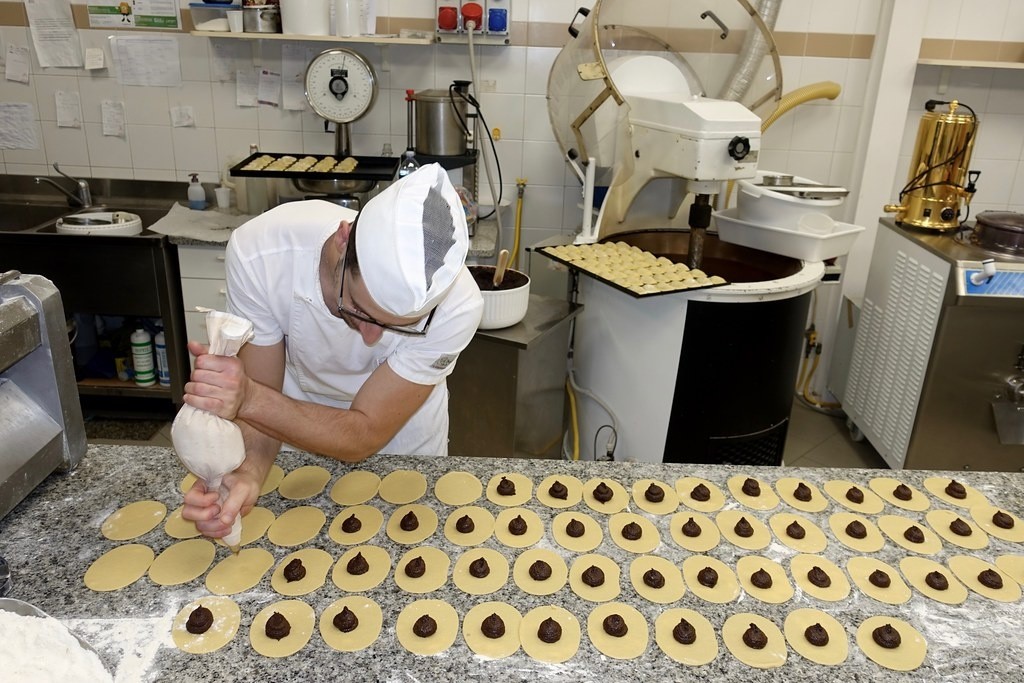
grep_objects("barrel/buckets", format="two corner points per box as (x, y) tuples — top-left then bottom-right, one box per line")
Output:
(55, 212), (143, 235)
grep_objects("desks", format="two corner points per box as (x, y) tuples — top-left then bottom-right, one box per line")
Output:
(448, 294), (585, 456)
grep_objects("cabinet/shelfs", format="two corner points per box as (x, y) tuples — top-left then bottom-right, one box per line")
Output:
(178, 242), (235, 372)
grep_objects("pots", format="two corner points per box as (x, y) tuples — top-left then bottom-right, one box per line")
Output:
(974, 210), (1022, 257)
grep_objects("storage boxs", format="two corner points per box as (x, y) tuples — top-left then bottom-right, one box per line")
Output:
(189, 3), (240, 33)
(711, 209), (865, 263)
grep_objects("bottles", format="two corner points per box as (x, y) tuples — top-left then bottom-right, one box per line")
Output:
(155, 328), (171, 387)
(130, 327), (156, 387)
(367, 143), (397, 200)
(246, 143), (270, 215)
(399, 151), (420, 179)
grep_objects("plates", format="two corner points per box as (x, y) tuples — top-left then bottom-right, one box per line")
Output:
(202, 0), (233, 4)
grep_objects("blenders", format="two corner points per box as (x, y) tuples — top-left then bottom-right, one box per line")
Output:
(547, 1), (827, 466)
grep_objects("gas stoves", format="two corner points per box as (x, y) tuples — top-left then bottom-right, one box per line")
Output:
(839, 216), (1023, 471)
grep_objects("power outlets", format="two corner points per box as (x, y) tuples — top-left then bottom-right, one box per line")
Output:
(487, 8), (510, 36)
(436, 5), (459, 34)
(461, 3), (485, 34)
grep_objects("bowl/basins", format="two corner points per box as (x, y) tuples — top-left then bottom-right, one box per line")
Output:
(186, 2), (241, 31)
(465, 264), (531, 331)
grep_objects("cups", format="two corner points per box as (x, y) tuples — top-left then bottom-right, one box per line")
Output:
(242, 5), (279, 33)
(226, 10), (244, 33)
(214, 188), (231, 209)
